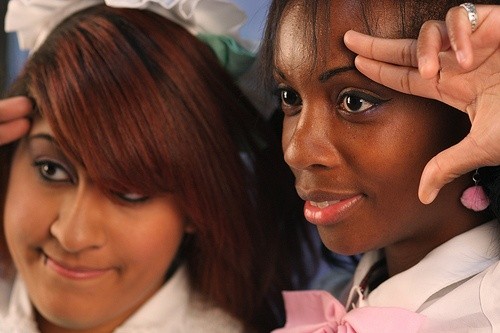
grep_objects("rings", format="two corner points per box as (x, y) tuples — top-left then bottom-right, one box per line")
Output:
(460, 2), (478, 33)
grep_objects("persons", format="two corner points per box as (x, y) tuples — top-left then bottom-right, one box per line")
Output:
(0, 0), (318, 333)
(256, 0), (500, 333)
